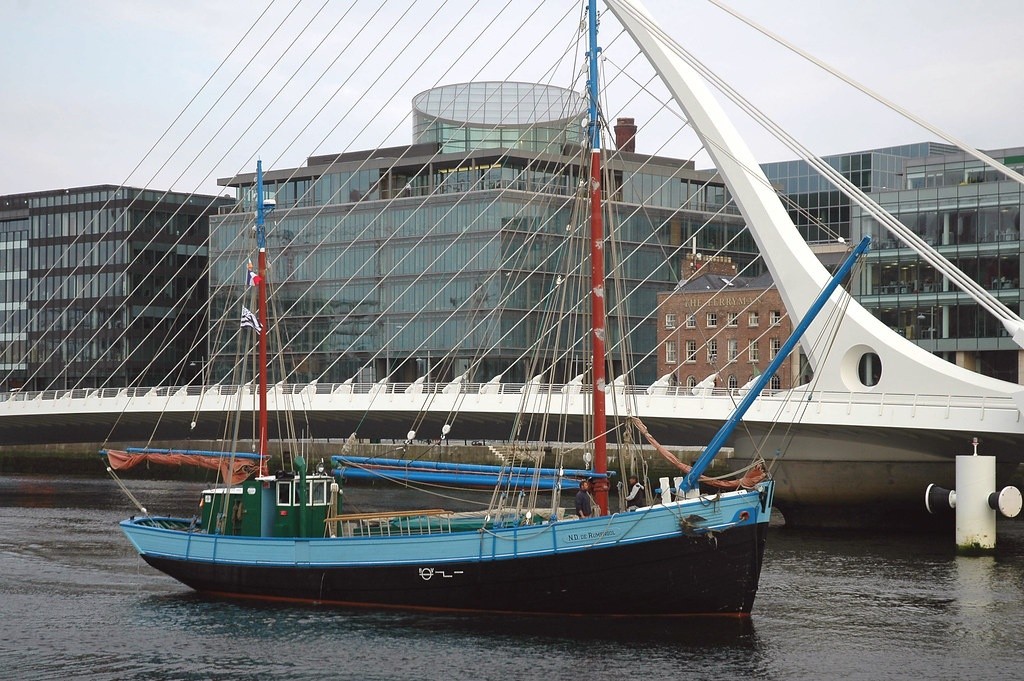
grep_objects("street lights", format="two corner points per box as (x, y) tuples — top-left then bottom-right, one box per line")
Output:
(917, 307), (936, 354)
(416, 351), (433, 394)
(190, 357), (204, 386)
(382, 317), (402, 381)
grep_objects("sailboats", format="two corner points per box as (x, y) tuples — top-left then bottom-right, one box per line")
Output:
(100, 0), (871, 632)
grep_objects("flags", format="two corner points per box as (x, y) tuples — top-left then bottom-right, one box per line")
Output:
(247, 269), (262, 286)
(241, 307), (264, 336)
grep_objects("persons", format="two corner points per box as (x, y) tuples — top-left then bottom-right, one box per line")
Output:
(626, 477), (645, 511)
(575, 481), (591, 519)
